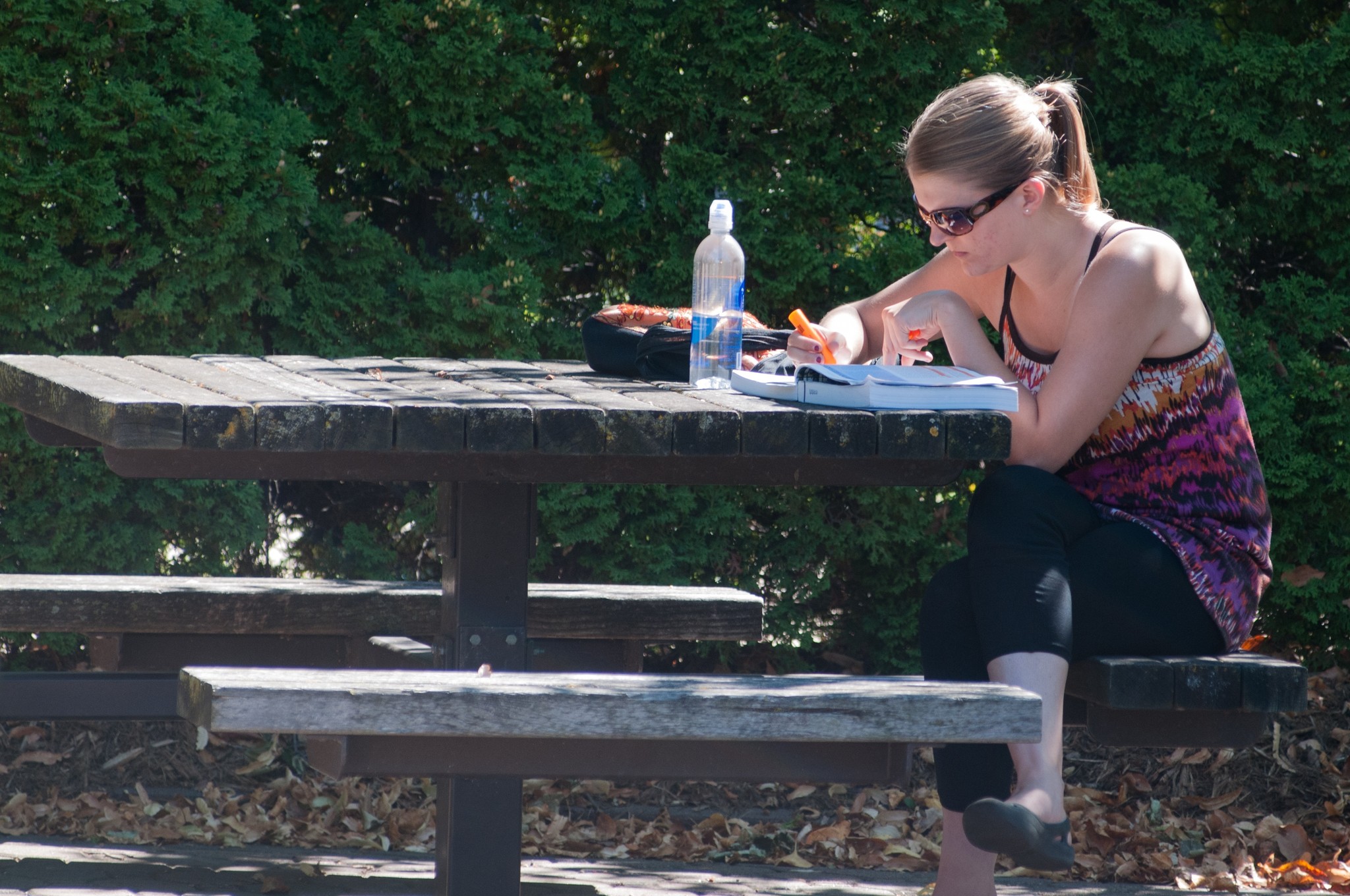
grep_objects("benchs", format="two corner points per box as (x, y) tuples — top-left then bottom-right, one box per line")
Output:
(163, 656), (1047, 790)
(1070, 647), (1311, 750)
(0, 573), (769, 725)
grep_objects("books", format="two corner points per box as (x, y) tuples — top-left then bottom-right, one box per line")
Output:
(731, 363), (1019, 413)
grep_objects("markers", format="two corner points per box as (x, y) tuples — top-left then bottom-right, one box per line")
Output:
(788, 308), (836, 365)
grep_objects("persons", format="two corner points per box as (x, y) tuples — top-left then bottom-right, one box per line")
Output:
(788, 71), (1274, 896)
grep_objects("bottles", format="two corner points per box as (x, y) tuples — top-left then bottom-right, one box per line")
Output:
(689, 198), (745, 392)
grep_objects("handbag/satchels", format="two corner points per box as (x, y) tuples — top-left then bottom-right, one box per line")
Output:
(582, 303), (794, 379)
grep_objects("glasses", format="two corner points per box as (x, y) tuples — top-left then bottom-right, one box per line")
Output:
(913, 173), (1033, 236)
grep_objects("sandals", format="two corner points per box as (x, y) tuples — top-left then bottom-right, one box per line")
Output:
(962, 798), (1075, 872)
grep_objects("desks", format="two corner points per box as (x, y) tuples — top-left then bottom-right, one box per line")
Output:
(0, 352), (1017, 896)
